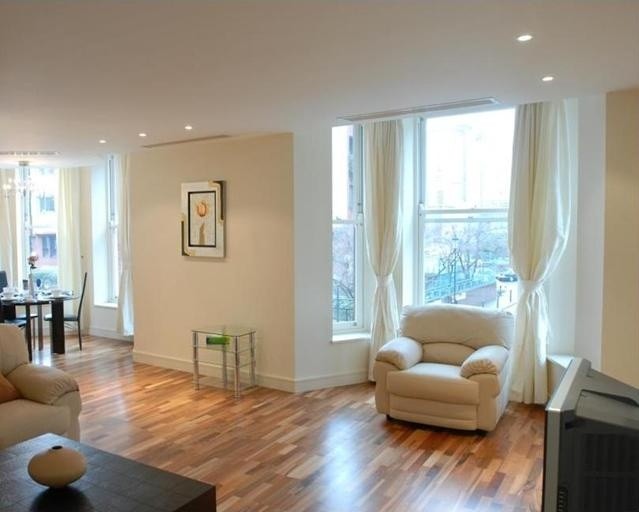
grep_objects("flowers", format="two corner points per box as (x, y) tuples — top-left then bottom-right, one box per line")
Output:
(27, 255), (38, 274)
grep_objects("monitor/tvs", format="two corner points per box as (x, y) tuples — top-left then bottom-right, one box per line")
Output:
(541, 358), (639, 512)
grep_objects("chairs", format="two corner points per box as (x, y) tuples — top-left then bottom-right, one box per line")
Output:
(372, 303), (515, 437)
(0, 265), (87, 362)
(0, 323), (82, 451)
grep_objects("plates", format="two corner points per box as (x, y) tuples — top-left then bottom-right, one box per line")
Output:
(0, 286), (69, 301)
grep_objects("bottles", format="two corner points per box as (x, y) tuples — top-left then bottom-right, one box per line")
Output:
(206, 336), (231, 344)
(23, 279), (28, 290)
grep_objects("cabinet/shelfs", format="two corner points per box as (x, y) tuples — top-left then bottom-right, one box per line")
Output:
(191, 324), (257, 400)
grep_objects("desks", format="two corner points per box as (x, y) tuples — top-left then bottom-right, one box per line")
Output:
(0, 433), (216, 512)
